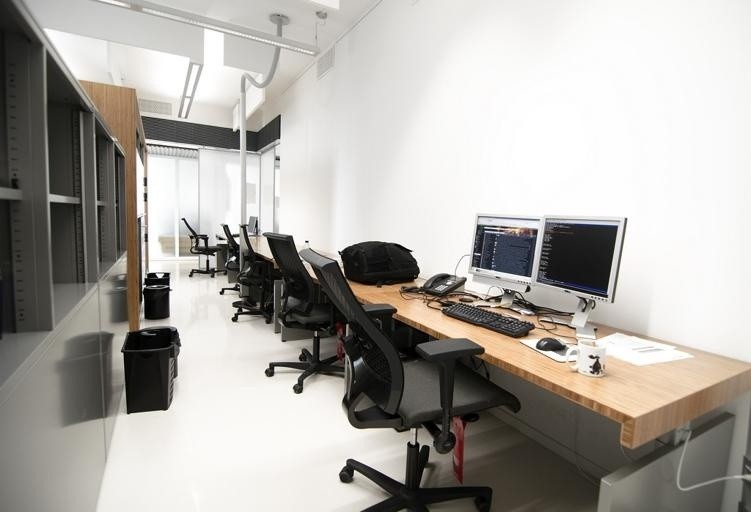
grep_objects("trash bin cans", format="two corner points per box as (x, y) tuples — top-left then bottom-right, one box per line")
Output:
(143, 273), (170, 320)
(121, 326), (180, 414)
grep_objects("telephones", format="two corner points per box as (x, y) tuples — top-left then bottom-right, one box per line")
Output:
(422, 273), (466, 296)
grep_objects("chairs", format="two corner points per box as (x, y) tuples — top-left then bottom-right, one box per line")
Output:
(219, 224), (241, 294)
(297, 246), (522, 512)
(182, 216), (220, 278)
(233, 219), (260, 238)
(233, 224), (281, 324)
(263, 231), (345, 394)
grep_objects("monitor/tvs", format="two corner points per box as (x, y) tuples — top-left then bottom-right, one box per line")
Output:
(467, 212), (541, 315)
(247, 216), (259, 236)
(532, 215), (629, 339)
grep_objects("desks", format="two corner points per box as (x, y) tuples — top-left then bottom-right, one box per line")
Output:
(303, 262), (428, 394)
(244, 244), (337, 335)
(355, 293), (751, 512)
(216, 234), (270, 298)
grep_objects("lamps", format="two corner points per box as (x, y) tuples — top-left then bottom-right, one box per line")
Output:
(94, 0), (328, 59)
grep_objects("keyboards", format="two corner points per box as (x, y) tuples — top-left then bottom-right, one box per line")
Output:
(441, 304), (535, 338)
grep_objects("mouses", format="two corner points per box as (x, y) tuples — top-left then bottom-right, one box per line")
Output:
(536, 337), (568, 351)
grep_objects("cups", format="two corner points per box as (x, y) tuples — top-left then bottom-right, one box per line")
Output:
(566, 339), (608, 378)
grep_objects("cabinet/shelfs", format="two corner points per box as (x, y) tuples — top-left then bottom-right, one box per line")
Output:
(78, 78), (150, 332)
(0, 0), (128, 512)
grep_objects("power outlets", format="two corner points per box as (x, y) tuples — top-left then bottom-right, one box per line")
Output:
(738, 455), (750, 511)
